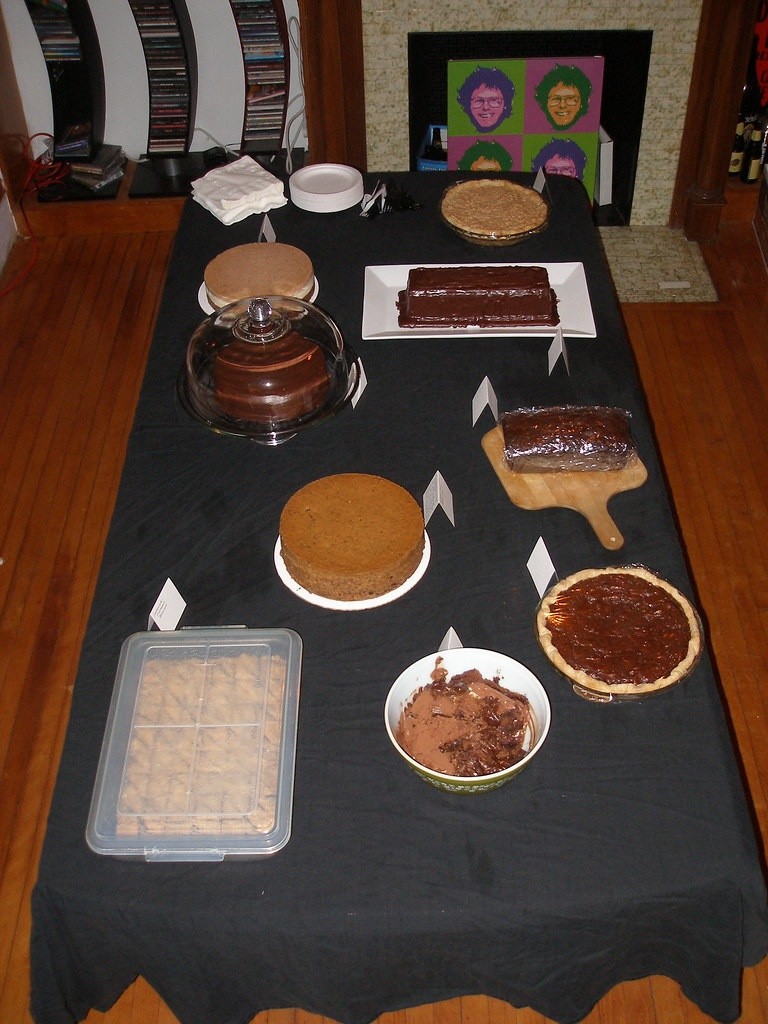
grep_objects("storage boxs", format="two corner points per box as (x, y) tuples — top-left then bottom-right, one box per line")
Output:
(594, 125), (613, 206)
(85, 624), (303, 863)
(417, 124), (448, 171)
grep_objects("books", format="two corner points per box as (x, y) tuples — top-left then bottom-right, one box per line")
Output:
(130, 0), (188, 154)
(230, 0), (286, 141)
(28, 7), (83, 62)
(54, 119), (125, 192)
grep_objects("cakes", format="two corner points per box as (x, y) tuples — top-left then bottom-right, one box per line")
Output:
(398, 267), (560, 328)
(211, 332), (330, 424)
(205, 242), (315, 321)
(278, 471), (424, 602)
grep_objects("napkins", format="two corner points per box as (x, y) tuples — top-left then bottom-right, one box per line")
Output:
(191, 155), (287, 225)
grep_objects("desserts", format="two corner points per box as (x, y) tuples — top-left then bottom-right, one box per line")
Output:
(396, 669), (532, 777)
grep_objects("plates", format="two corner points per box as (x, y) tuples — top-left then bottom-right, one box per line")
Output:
(198, 275), (319, 326)
(534, 562), (704, 703)
(274, 529), (431, 611)
(362, 261), (597, 340)
(289, 163), (364, 213)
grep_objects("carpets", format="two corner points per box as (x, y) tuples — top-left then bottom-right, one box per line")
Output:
(598, 223), (719, 305)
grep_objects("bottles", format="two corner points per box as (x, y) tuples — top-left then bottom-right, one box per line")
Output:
(728, 113), (768, 184)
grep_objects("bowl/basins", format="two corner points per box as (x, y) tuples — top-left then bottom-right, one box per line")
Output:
(438, 179), (552, 247)
(384, 647), (551, 795)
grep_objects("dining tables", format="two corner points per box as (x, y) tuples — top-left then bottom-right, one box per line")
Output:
(31, 169), (768, 1024)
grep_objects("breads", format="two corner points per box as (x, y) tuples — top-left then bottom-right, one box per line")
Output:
(502, 404), (633, 474)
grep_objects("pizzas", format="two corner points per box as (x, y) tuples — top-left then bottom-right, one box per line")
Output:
(441, 178), (548, 236)
(536, 567), (699, 693)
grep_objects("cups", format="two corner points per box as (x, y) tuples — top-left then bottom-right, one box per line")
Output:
(164, 158), (182, 176)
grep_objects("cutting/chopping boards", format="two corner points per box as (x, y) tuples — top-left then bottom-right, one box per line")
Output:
(481, 422), (647, 550)
